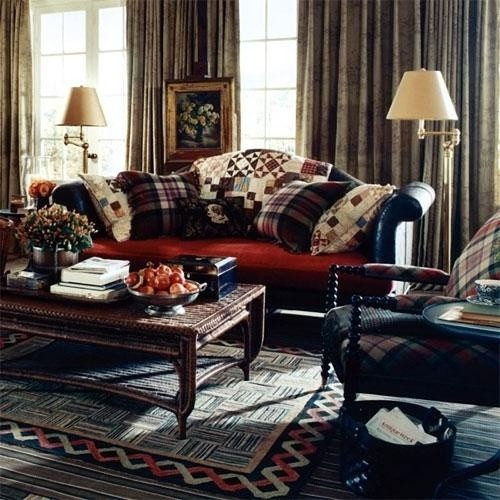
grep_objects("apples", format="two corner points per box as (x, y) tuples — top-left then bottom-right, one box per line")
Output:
(124, 264), (198, 297)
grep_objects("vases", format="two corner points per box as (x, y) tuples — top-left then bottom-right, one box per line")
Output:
(37, 194), (50, 213)
(31, 245), (79, 287)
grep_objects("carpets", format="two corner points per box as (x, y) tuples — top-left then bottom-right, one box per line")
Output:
(0, 328), (354, 500)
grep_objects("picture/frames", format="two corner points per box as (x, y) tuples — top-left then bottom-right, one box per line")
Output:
(162, 74), (237, 170)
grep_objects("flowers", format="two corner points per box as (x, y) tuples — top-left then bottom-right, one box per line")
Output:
(28, 178), (56, 199)
(14, 203), (99, 254)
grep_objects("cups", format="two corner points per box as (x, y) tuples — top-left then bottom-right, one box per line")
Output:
(474, 279), (499, 302)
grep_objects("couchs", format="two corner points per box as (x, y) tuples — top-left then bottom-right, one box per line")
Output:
(49, 149), (435, 338)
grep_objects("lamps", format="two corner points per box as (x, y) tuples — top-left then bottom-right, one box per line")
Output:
(62, 85), (107, 174)
(386, 69), (461, 296)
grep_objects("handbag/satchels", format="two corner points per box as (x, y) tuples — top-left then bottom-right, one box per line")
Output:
(337, 400), (457, 499)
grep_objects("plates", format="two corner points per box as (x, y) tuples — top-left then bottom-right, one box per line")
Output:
(466, 294), (499, 307)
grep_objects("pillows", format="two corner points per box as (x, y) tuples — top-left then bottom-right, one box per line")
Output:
(172, 196), (247, 241)
(444, 208), (500, 300)
(117, 169), (201, 241)
(78, 175), (133, 243)
(310, 183), (395, 256)
(247, 179), (359, 254)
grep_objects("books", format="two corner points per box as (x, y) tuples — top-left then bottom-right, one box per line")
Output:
(459, 305), (500, 324)
(48, 256), (131, 300)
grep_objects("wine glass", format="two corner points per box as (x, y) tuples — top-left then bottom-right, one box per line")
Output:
(126, 278), (208, 317)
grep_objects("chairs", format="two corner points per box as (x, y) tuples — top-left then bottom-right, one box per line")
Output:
(323, 261), (500, 441)
